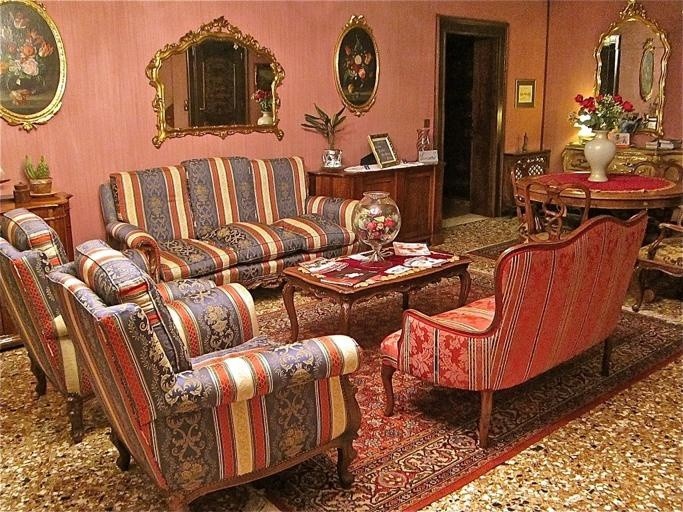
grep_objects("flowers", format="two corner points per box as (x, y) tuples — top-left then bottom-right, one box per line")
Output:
(0, 10), (55, 106)
(567, 92), (635, 131)
(342, 33), (373, 97)
(250, 89), (273, 112)
(353, 210), (400, 245)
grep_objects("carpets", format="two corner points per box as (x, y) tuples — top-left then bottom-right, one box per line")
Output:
(466, 233), (532, 260)
(248, 265), (683, 510)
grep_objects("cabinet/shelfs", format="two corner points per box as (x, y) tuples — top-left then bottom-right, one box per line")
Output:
(561, 143), (683, 216)
(0, 190), (75, 351)
(502, 147), (551, 219)
(307, 159), (446, 245)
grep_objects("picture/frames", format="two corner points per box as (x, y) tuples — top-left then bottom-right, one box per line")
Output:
(614, 132), (631, 146)
(0, 0), (67, 132)
(255, 62), (275, 92)
(366, 133), (439, 169)
(514, 80), (536, 109)
(332, 14), (381, 117)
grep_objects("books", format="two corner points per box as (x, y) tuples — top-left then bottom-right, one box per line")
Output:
(392, 240), (431, 258)
(330, 259), (349, 272)
(298, 256), (339, 275)
(645, 137), (681, 149)
(320, 266), (376, 287)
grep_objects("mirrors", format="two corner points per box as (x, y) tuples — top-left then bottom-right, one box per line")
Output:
(639, 38), (656, 102)
(592, 0), (671, 138)
(144, 14), (285, 149)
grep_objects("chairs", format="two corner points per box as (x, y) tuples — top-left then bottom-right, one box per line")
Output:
(513, 161), (683, 312)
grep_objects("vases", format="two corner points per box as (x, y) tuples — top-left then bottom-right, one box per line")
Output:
(257, 111), (273, 125)
(352, 191), (401, 268)
(583, 130), (616, 183)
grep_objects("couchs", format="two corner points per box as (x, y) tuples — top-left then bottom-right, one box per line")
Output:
(98, 182), (368, 289)
(377, 209), (650, 448)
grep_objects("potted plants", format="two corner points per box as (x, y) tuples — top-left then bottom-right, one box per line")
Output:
(23, 154), (53, 193)
(299, 101), (347, 169)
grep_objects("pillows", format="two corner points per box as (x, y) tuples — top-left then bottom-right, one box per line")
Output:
(109, 156), (309, 243)
